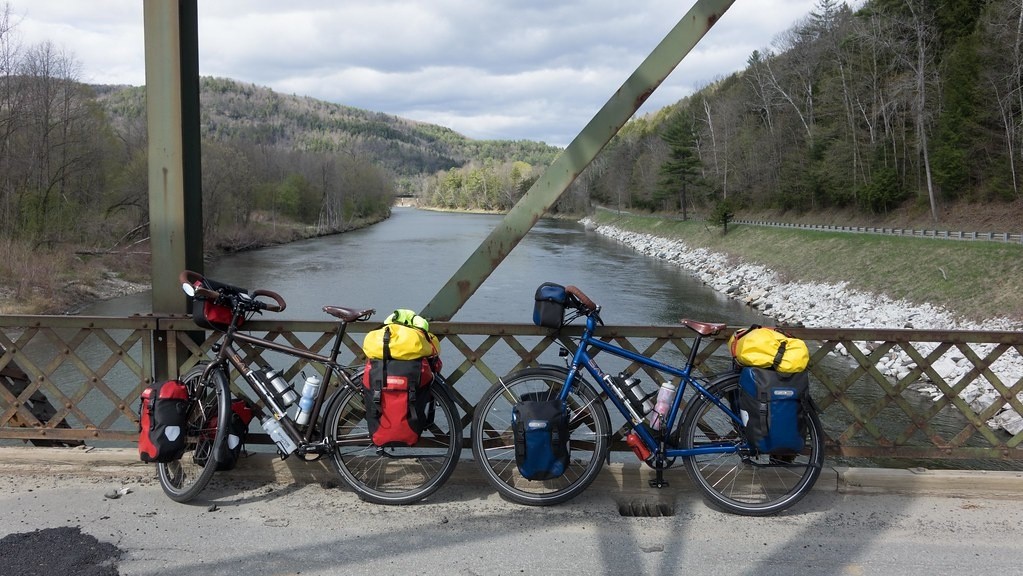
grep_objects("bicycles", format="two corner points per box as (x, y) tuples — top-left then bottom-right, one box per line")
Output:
(468, 282), (826, 519)
(161, 270), (466, 507)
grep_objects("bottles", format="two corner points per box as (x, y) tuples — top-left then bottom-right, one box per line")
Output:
(257, 413), (297, 457)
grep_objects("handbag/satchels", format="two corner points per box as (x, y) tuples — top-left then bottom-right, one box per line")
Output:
(511, 389), (572, 482)
(533, 281), (565, 327)
(737, 364), (809, 456)
(191, 278), (248, 330)
(728, 323), (809, 374)
(135, 378), (187, 463)
(363, 323), (440, 361)
(363, 357), (434, 448)
(194, 398), (255, 471)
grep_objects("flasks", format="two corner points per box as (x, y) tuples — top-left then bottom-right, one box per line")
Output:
(260, 365), (297, 408)
(623, 429), (652, 460)
(619, 372), (654, 415)
(647, 380), (675, 429)
(294, 375), (319, 426)
